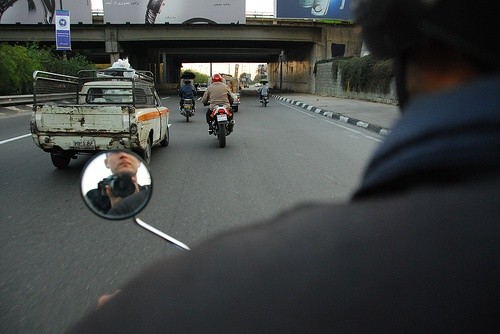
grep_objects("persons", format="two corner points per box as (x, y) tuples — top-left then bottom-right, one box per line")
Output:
(86, 152), (151, 214)
(63, 0), (500, 334)
(256, 82), (269, 101)
(179, 80), (197, 111)
(202, 73), (234, 133)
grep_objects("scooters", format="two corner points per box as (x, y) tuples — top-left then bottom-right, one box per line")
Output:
(261, 96), (270, 106)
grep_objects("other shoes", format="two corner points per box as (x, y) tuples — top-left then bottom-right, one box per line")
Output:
(266, 100), (268, 102)
(209, 126), (213, 134)
(228, 127), (233, 132)
(179, 106), (183, 113)
(260, 100), (262, 101)
(191, 110), (194, 115)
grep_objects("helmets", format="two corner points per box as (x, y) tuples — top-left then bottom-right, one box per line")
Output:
(212, 74), (222, 82)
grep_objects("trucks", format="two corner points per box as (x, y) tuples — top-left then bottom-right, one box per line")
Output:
(28, 69), (170, 163)
(207, 74), (241, 112)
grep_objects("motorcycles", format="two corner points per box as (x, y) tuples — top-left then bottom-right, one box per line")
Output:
(182, 98), (196, 122)
(207, 104), (237, 148)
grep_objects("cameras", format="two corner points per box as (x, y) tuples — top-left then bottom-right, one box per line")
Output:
(98, 173), (137, 198)
